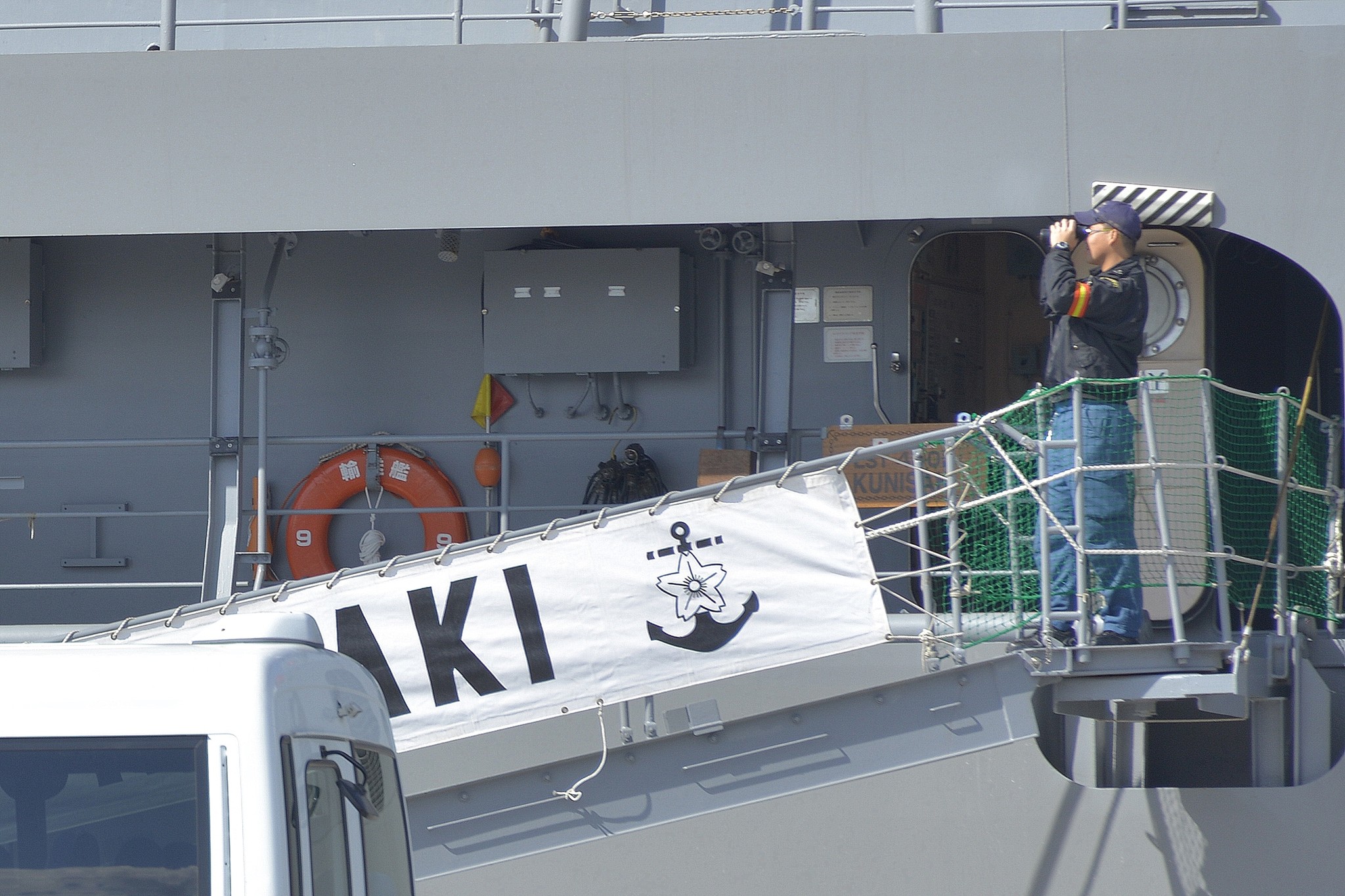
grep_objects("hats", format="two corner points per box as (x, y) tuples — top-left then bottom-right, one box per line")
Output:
(1073, 200), (1142, 241)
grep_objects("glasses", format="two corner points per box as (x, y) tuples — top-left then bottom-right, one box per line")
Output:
(1085, 226), (1113, 237)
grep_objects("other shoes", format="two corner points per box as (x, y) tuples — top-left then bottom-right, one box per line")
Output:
(1006, 624), (1078, 653)
(1091, 630), (1140, 646)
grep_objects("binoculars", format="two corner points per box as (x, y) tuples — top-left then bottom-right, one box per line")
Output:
(1040, 225), (1089, 242)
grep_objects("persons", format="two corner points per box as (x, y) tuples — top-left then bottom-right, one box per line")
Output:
(1005, 201), (1155, 653)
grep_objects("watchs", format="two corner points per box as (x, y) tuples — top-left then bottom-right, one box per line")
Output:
(1052, 240), (1073, 256)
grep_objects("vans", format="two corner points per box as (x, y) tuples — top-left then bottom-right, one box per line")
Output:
(1, 611), (423, 896)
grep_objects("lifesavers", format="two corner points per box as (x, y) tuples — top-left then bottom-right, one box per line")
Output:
(286, 446), (471, 579)
